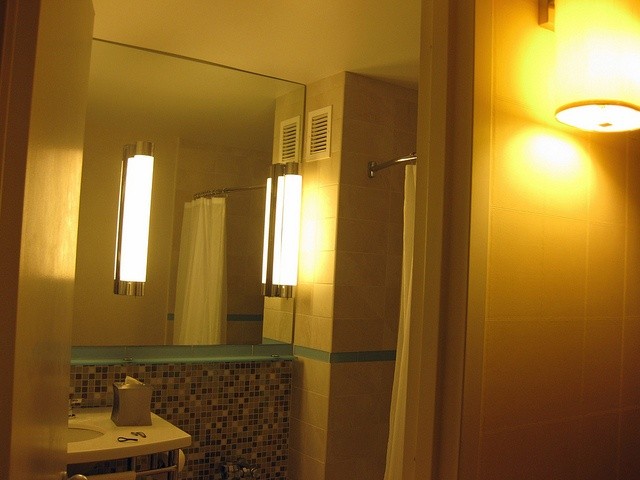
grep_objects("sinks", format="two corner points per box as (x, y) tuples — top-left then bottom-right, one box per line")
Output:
(67, 427), (105, 443)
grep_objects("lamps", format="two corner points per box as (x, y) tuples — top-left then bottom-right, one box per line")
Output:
(276, 160), (303, 299)
(117, 138), (155, 297)
(259, 163), (281, 298)
(113, 141), (136, 296)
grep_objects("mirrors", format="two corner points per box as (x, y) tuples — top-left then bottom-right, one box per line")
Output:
(73, 36), (308, 349)
